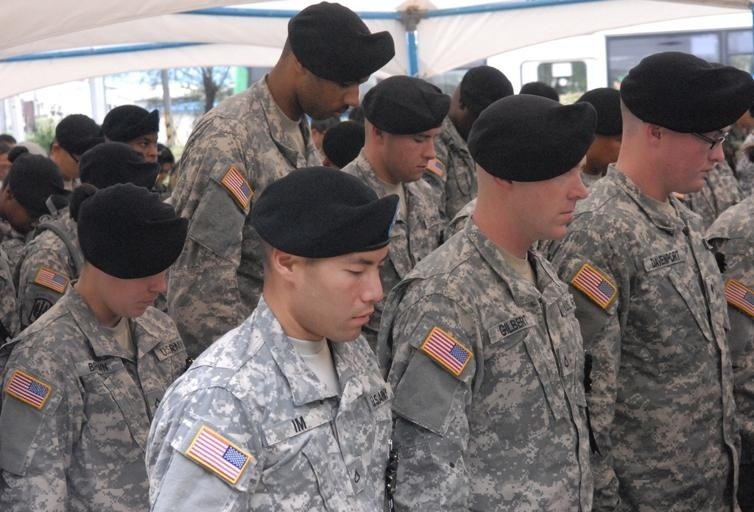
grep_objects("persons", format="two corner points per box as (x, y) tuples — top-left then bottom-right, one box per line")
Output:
(143, 167), (396, 512)
(2, 105), (190, 512)
(162, 1), (395, 355)
(306, 50), (754, 512)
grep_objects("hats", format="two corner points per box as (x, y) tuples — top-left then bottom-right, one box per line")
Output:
(348, 107), (364, 122)
(621, 51), (754, 132)
(289, 3), (396, 83)
(461, 66), (514, 116)
(249, 166), (399, 257)
(520, 82), (560, 102)
(574, 88), (622, 135)
(324, 121), (365, 168)
(361, 76), (452, 135)
(8, 105), (189, 278)
(467, 94), (597, 180)
